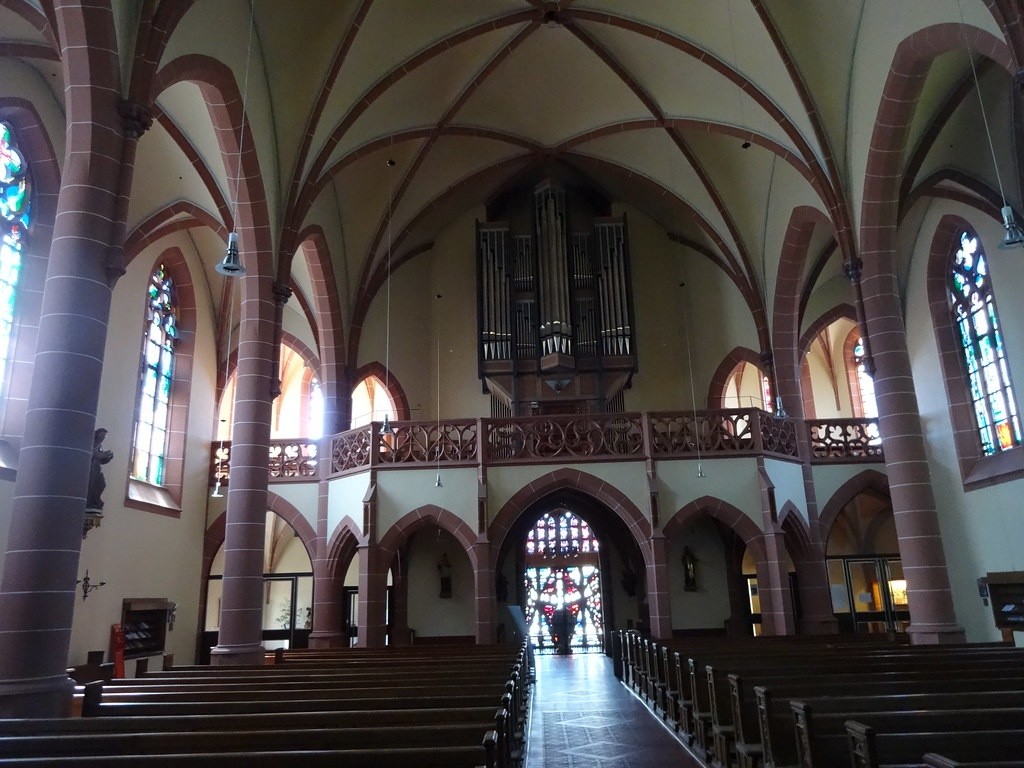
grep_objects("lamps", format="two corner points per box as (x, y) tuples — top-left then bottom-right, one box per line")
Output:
(431, 290), (448, 489)
(210, 0), (258, 280)
(676, 275), (709, 481)
(769, 318), (792, 421)
(949, 0), (1024, 249)
(211, 278), (236, 500)
(371, 156), (402, 437)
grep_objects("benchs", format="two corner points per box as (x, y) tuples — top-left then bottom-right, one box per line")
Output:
(0, 634), (540, 768)
(612, 627), (1024, 768)
